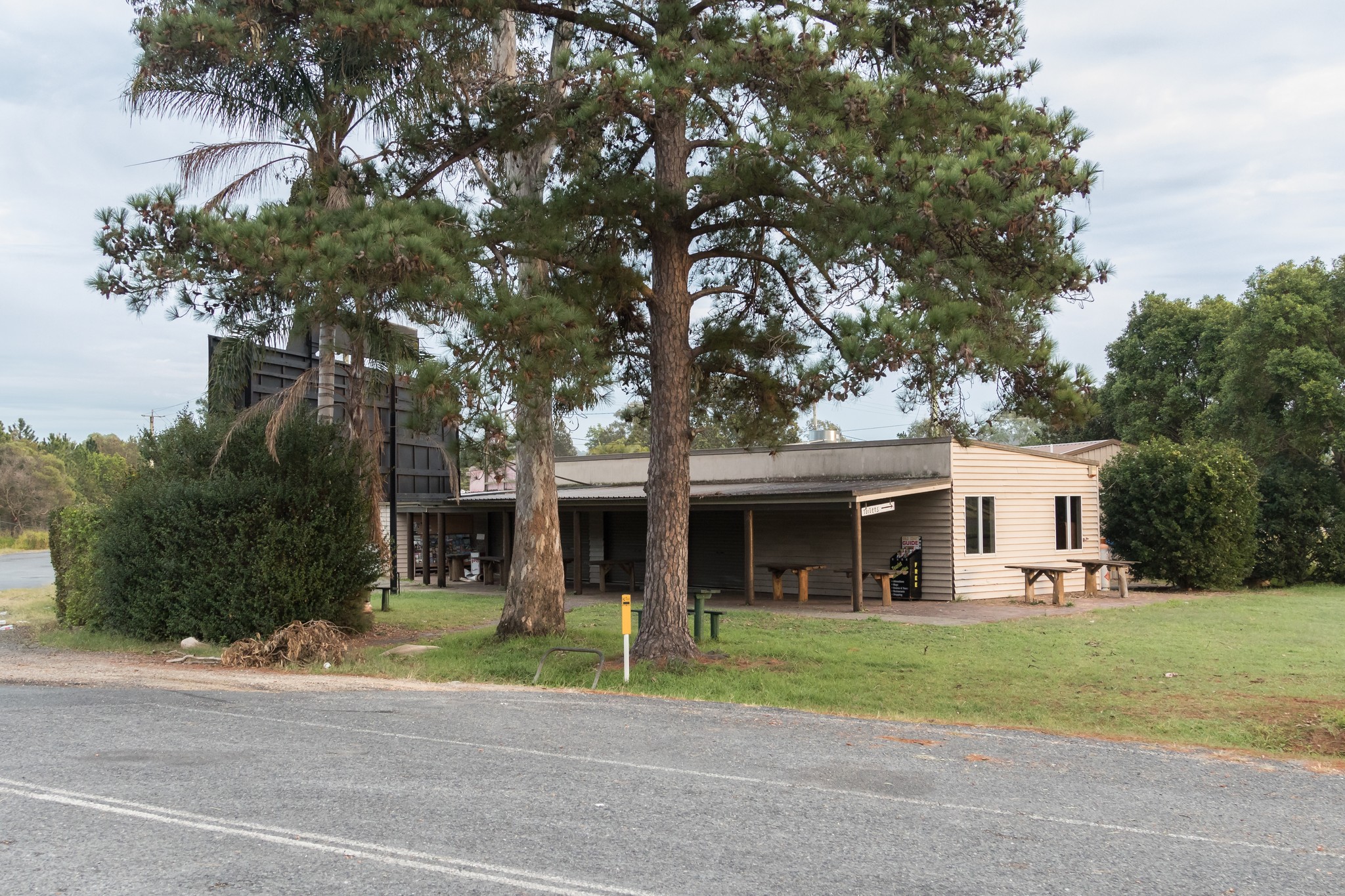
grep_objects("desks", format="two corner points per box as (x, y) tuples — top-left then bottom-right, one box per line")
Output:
(639, 584), (721, 645)
(833, 567), (908, 605)
(1067, 558), (1142, 598)
(1005, 565), (1082, 607)
(756, 562), (827, 603)
(470, 556), (506, 586)
(445, 554), (471, 582)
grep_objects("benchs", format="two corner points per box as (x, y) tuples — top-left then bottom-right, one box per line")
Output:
(371, 586), (398, 612)
(630, 609), (696, 633)
(687, 608), (727, 644)
